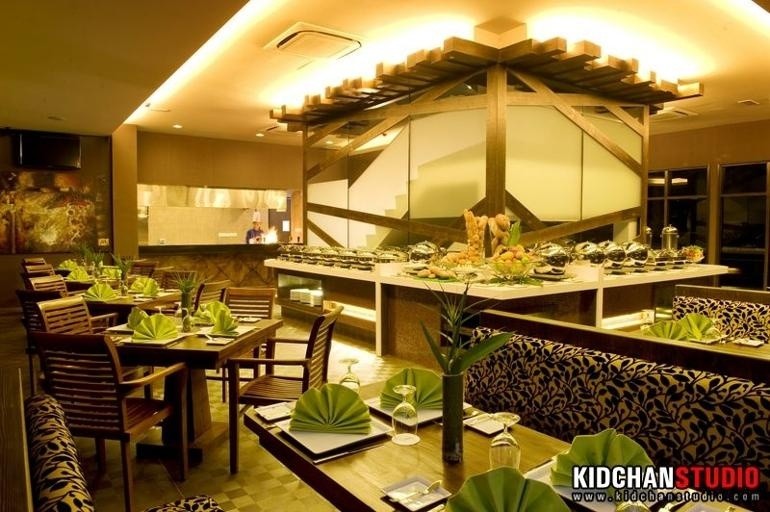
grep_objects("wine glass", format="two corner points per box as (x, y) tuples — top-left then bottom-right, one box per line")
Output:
(485, 412), (524, 472)
(390, 384), (418, 445)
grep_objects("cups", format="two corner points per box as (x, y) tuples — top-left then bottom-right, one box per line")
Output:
(640, 309), (655, 329)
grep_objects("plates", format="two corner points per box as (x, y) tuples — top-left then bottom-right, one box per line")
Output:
(693, 333), (765, 348)
(254, 401), (294, 421)
(106, 316), (271, 346)
(363, 396), (472, 428)
(275, 240), (685, 280)
(522, 457), (673, 512)
(289, 288), (322, 307)
(275, 406), (393, 457)
(463, 413), (506, 434)
(381, 475), (452, 512)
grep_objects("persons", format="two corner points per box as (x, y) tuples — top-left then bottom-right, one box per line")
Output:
(245, 221), (267, 245)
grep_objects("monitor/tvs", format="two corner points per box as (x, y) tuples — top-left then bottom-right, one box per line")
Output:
(20, 132), (82, 171)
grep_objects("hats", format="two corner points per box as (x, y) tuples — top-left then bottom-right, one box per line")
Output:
(252, 212), (260, 223)
